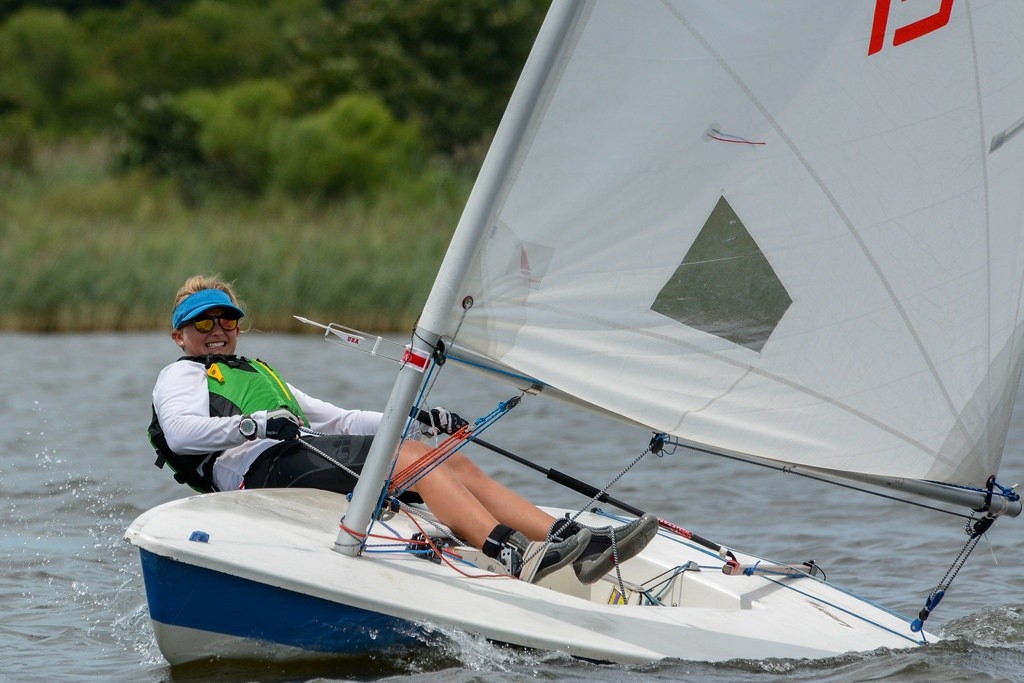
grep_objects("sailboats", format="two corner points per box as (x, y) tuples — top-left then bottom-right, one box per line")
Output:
(122, 0), (1024, 661)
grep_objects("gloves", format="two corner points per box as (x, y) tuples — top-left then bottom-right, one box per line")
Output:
(418, 405), (469, 438)
(266, 406), (303, 443)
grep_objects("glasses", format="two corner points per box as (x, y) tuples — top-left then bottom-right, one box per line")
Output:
(181, 313), (238, 333)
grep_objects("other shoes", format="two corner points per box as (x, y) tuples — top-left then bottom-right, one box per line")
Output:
(566, 512), (659, 584)
(501, 525), (593, 585)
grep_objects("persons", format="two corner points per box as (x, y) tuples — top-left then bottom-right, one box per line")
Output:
(146, 274), (661, 585)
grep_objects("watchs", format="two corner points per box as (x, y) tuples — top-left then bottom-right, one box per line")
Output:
(239, 413), (258, 441)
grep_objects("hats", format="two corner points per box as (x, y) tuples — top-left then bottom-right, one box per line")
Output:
(172, 288), (245, 330)
